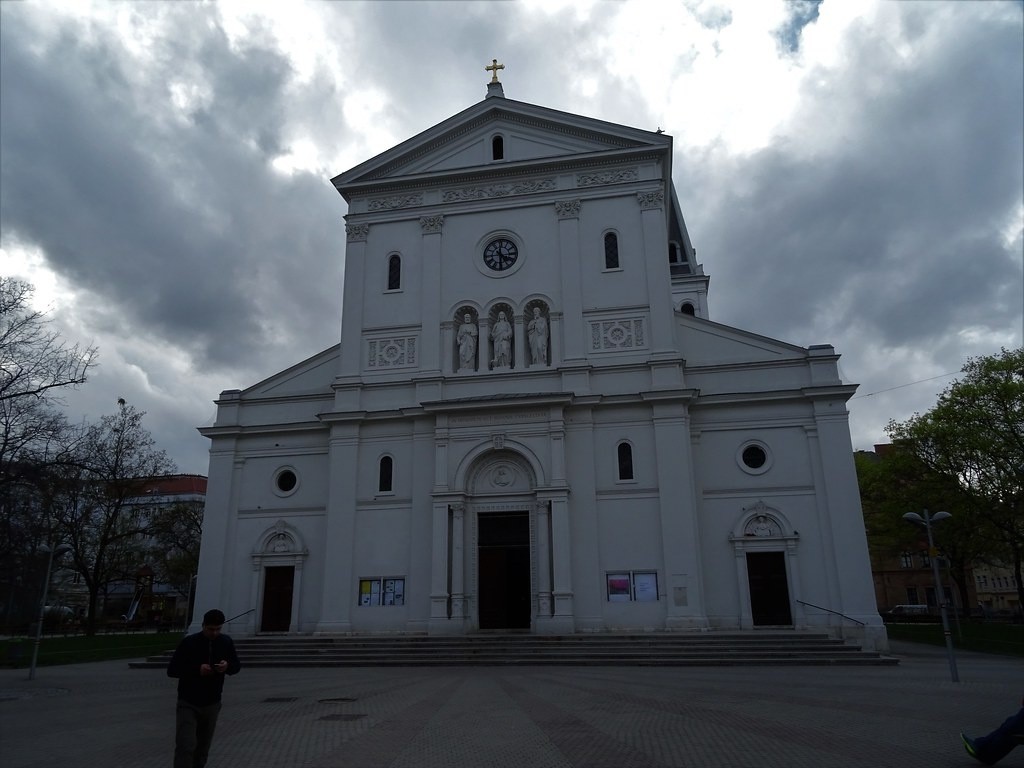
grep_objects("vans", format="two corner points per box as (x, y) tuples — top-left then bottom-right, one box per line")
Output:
(887, 604), (928, 616)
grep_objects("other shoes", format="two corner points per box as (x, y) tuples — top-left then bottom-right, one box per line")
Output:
(961, 733), (979, 757)
(965, 745), (990, 767)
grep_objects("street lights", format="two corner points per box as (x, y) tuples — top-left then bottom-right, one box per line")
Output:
(902, 508), (961, 683)
(28, 540), (60, 680)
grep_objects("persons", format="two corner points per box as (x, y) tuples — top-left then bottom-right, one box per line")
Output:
(527, 307), (550, 366)
(491, 310), (513, 370)
(166, 608), (241, 768)
(457, 313), (478, 371)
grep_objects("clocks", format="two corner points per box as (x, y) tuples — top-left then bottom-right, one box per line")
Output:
(483, 239), (519, 272)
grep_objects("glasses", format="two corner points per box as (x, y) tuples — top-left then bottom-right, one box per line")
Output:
(207, 626), (222, 632)
